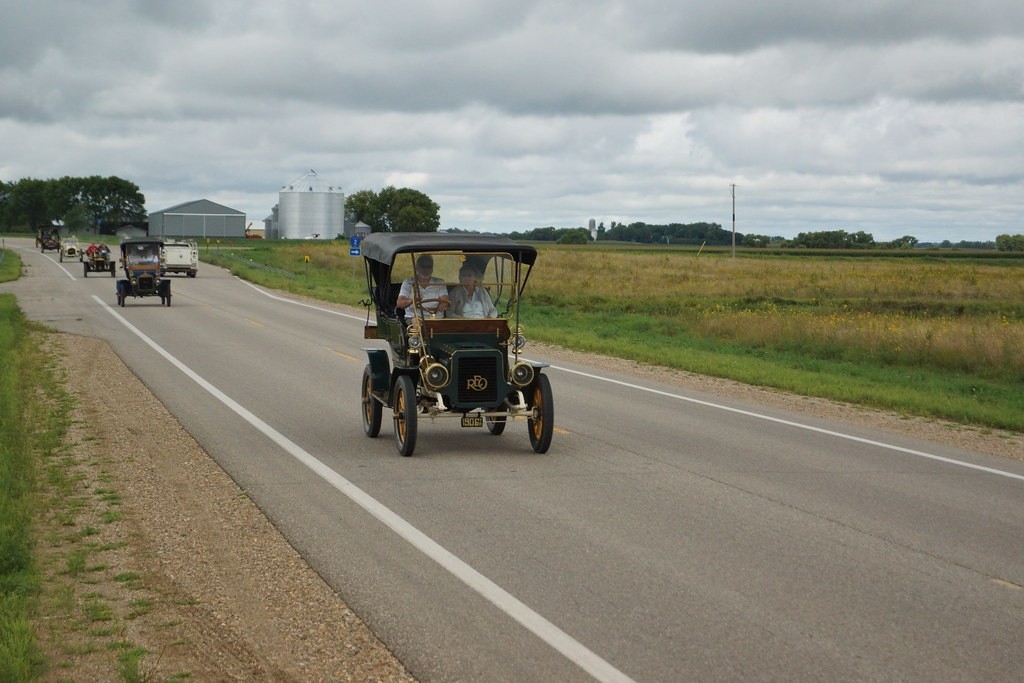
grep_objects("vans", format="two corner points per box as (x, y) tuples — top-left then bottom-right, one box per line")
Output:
(158, 237), (199, 278)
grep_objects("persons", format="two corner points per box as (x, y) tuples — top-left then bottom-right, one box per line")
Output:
(436, 266), (500, 320)
(395, 256), (448, 327)
(87, 242), (98, 254)
(128, 247), (142, 262)
(98, 242), (110, 259)
(141, 249), (158, 264)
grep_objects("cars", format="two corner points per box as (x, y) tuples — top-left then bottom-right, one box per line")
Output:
(59, 237), (83, 263)
(82, 248), (116, 278)
(35, 225), (61, 254)
(116, 237), (172, 308)
(359, 231), (554, 456)
